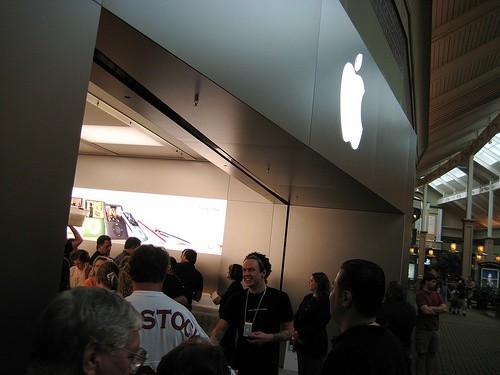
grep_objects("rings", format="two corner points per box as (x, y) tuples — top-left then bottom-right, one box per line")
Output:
(292, 337), (295, 340)
(183, 300), (184, 302)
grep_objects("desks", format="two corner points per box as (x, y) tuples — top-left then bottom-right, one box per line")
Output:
(191, 292), (220, 337)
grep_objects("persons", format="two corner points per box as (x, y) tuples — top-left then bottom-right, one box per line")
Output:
(161, 249), (203, 312)
(436, 277), (500, 320)
(81, 256), (114, 288)
(26, 286), (149, 375)
(114, 237), (141, 266)
(210, 264), (244, 370)
(123, 245), (239, 375)
(95, 261), (119, 293)
(72, 200), (138, 225)
(211, 252), (293, 375)
(64, 225), (83, 261)
(290, 272), (331, 375)
(89, 235), (112, 266)
(416, 273), (449, 375)
(69, 249), (91, 288)
(320, 259), (412, 375)
(375, 281), (417, 370)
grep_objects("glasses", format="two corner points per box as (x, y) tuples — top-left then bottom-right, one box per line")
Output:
(108, 343), (148, 371)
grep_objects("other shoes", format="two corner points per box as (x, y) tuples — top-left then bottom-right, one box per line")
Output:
(457, 311), (459, 314)
(452, 311), (455, 314)
(449, 308), (451, 312)
(462, 314), (466, 316)
